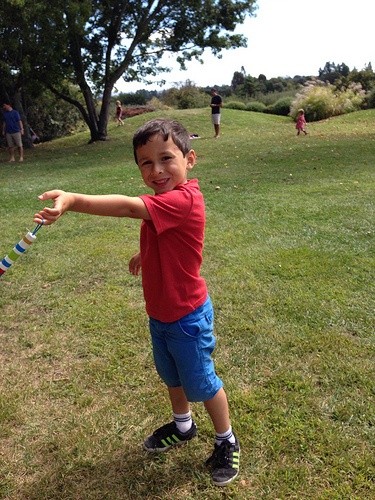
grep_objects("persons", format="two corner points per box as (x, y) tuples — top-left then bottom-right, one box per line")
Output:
(34, 116), (240, 486)
(28, 120), (40, 148)
(2, 100), (25, 163)
(294, 109), (309, 136)
(209, 88), (223, 138)
(115, 100), (125, 126)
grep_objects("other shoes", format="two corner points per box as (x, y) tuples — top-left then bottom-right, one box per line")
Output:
(143, 420), (197, 452)
(205, 432), (241, 487)
